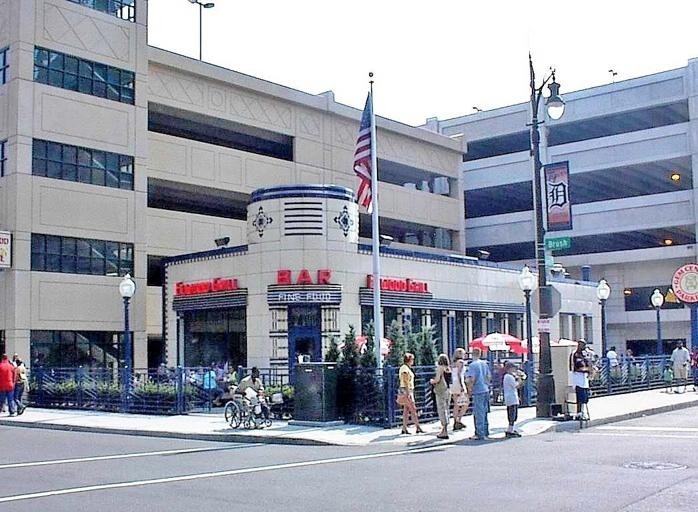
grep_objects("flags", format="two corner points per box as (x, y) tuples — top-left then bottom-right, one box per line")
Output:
(351, 92), (373, 213)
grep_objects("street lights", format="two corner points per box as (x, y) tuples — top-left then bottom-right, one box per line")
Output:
(649, 288), (664, 355)
(189, 0), (215, 59)
(518, 263), (536, 376)
(119, 272), (137, 409)
(597, 277), (610, 357)
(527, 54), (566, 420)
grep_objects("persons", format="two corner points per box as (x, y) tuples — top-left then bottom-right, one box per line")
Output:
(662, 340), (697, 394)
(569, 340), (592, 420)
(1, 353), (29, 416)
(192, 361), (238, 408)
(592, 346), (635, 366)
(234, 367), (271, 427)
(398, 348), (523, 441)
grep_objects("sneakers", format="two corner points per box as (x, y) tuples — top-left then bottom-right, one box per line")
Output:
(402, 422), (521, 440)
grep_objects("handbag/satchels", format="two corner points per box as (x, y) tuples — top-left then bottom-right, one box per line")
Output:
(449, 384), (462, 394)
(397, 389), (414, 405)
(568, 372), (588, 387)
(433, 378), (447, 394)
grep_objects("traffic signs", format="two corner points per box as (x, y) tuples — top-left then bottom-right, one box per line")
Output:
(545, 234), (570, 250)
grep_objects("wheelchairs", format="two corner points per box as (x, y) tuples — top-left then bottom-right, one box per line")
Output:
(224, 389), (273, 430)
